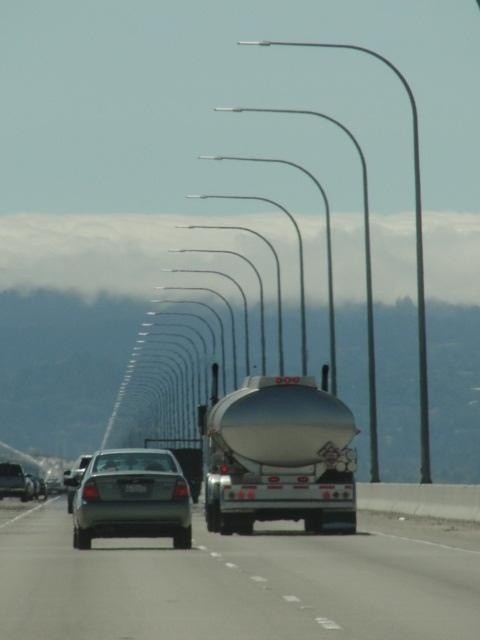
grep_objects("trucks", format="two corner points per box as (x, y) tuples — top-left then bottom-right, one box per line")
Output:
(197, 359), (362, 535)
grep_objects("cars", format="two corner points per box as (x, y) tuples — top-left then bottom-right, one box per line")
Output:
(0, 445), (93, 513)
(64, 448), (201, 549)
